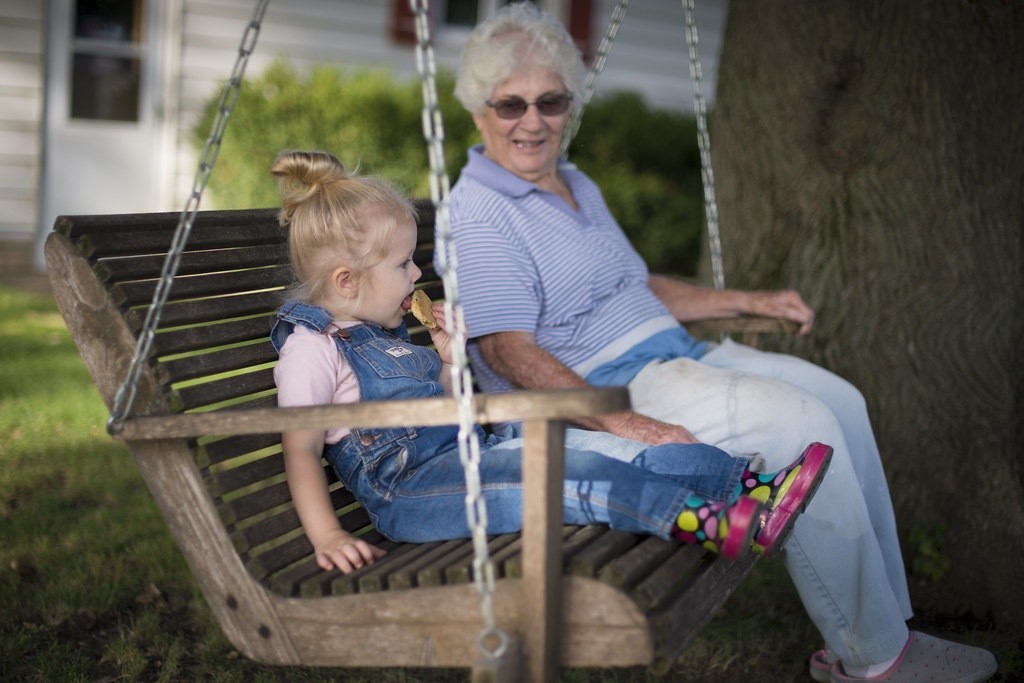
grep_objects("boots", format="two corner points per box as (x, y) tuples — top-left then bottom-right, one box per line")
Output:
(668, 442), (835, 564)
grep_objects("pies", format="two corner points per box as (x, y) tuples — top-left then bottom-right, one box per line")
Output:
(411, 290), (438, 329)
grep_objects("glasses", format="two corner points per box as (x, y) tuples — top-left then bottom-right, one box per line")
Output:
(484, 90), (574, 120)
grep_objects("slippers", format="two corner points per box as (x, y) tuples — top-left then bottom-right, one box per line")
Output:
(810, 629), (997, 683)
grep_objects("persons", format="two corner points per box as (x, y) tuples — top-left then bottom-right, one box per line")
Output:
(271, 150), (834, 575)
(433, 4), (1001, 683)
